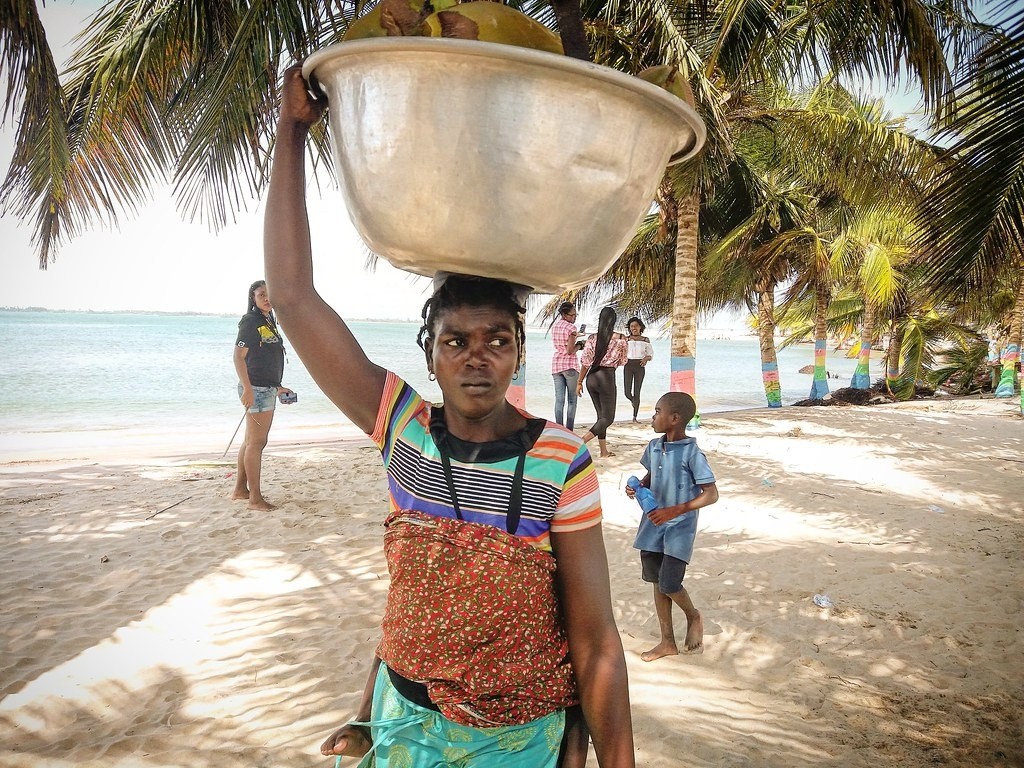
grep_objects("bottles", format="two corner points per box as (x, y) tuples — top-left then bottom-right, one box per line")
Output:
(627, 476), (659, 515)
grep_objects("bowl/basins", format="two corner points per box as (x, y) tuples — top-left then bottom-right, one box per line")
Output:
(300, 36), (708, 294)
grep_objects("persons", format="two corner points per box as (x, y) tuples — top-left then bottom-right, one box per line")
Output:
(552, 302), (589, 432)
(575, 307), (628, 457)
(624, 317), (653, 424)
(231, 279), (294, 512)
(625, 391), (720, 662)
(263, 54), (635, 768)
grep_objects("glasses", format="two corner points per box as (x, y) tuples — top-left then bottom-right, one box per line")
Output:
(564, 313), (579, 317)
